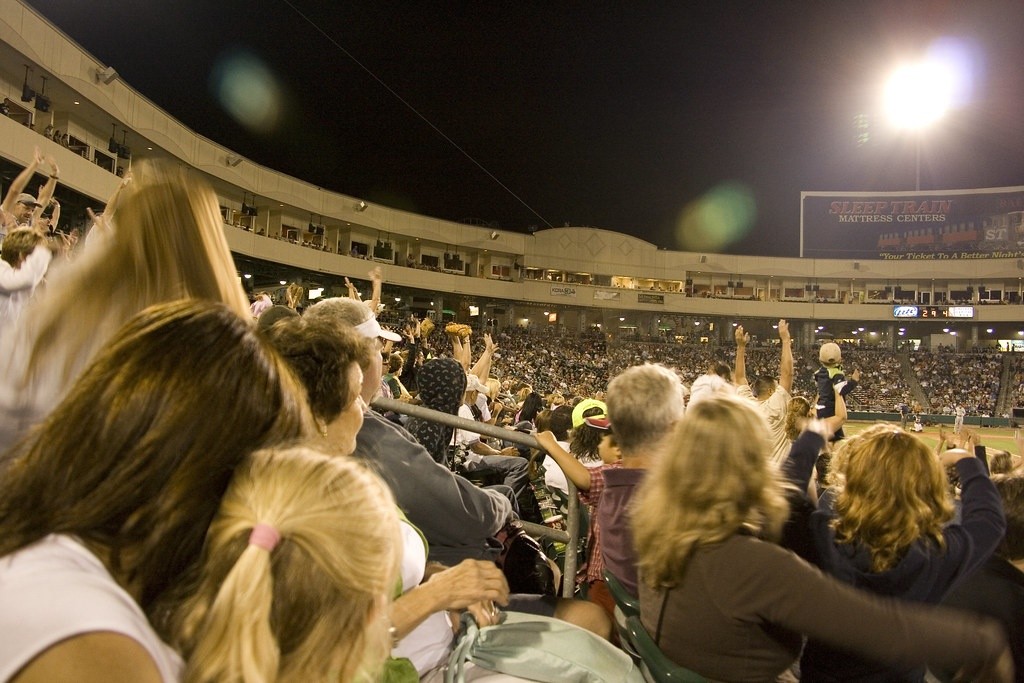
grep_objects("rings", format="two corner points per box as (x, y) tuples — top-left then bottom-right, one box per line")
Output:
(492, 612), (494, 616)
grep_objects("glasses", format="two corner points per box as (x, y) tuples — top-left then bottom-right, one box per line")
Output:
(383, 362), (391, 366)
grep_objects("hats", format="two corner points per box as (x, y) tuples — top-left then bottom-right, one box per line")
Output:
(466, 374), (490, 394)
(586, 417), (612, 429)
(572, 398), (609, 427)
(41, 205), (55, 218)
(820, 342), (840, 363)
(351, 313), (402, 344)
(18, 193), (42, 209)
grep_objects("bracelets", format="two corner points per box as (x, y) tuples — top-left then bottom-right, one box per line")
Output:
(389, 625), (398, 649)
(50, 175), (59, 179)
(462, 340), (470, 343)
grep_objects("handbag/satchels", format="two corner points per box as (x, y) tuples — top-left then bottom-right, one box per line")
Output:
(455, 607), (634, 683)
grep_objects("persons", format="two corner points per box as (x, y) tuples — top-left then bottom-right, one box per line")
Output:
(1, 95), (1024, 682)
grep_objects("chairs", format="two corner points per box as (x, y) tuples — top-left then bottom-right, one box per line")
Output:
(539, 466), (712, 683)
